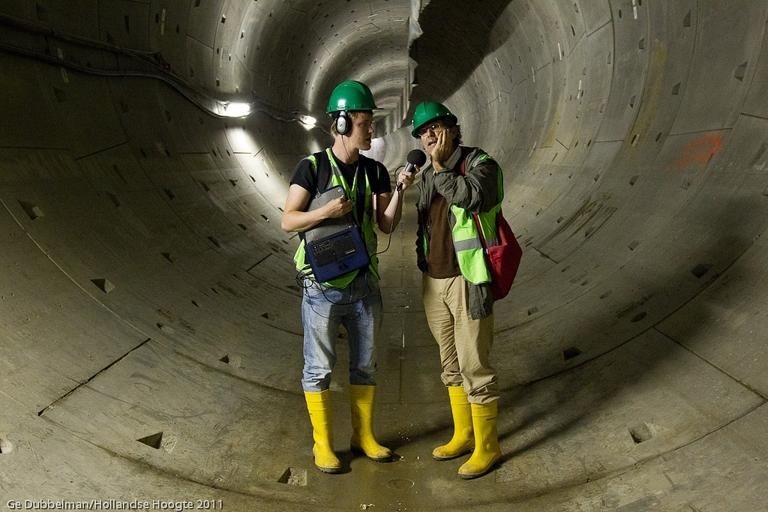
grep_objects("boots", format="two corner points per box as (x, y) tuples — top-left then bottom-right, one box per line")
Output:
(432, 384), (503, 479)
(348, 384), (393, 462)
(303, 388), (343, 473)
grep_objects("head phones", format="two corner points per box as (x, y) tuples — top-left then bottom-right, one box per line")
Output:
(335, 111), (352, 135)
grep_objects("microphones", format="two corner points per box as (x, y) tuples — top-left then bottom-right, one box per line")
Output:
(395, 149), (427, 192)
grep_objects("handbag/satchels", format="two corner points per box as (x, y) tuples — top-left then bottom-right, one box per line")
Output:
(301, 155), (371, 285)
(458, 147), (523, 300)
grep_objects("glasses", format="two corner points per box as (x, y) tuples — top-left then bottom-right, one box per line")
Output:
(417, 122), (446, 136)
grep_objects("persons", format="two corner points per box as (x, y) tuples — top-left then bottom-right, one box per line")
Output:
(281, 81), (421, 474)
(411, 97), (506, 479)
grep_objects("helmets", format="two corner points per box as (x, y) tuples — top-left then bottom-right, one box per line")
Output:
(411, 100), (458, 139)
(325, 80), (384, 114)
